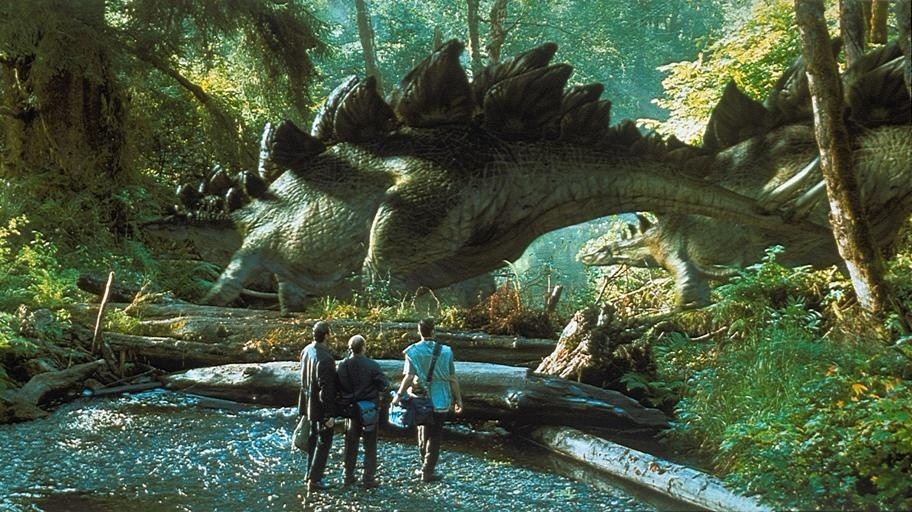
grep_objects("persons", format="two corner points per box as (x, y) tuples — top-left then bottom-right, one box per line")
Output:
(298, 321), (337, 490)
(337, 335), (389, 487)
(390, 318), (464, 482)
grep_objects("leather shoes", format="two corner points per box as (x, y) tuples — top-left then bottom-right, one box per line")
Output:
(364, 477), (380, 488)
(307, 481), (332, 492)
(415, 469), (422, 476)
(420, 470), (444, 483)
(343, 476), (359, 485)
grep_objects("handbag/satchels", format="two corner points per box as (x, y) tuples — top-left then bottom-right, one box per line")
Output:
(402, 342), (443, 428)
(291, 414), (316, 455)
(335, 360), (363, 419)
